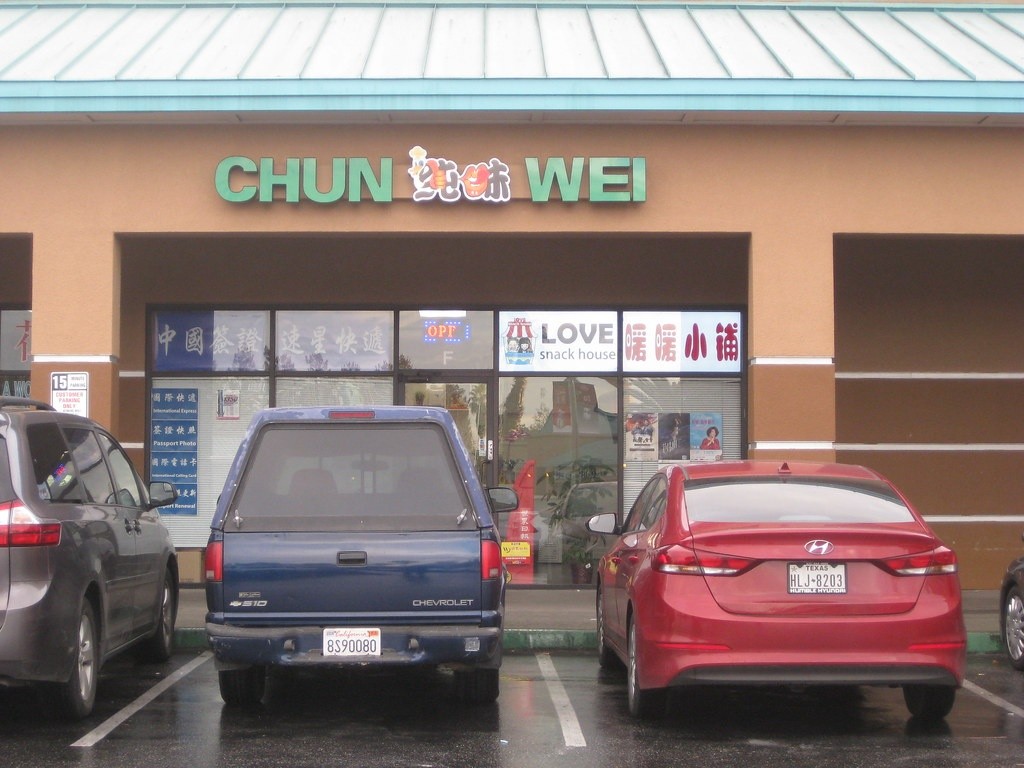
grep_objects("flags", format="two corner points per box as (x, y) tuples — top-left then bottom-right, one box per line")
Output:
(552, 381), (600, 430)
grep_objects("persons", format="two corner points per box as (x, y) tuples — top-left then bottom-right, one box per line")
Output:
(701, 427), (719, 450)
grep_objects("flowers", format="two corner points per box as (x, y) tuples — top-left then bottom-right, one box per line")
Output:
(498, 428), (528, 470)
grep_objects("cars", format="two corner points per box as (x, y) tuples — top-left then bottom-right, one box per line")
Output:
(207, 406), (519, 718)
(995, 554), (1024, 670)
(536, 482), (659, 584)
(586, 458), (967, 719)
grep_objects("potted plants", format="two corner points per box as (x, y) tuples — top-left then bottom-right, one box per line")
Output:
(535, 454), (617, 586)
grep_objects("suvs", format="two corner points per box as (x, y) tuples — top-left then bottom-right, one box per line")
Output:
(0, 392), (187, 722)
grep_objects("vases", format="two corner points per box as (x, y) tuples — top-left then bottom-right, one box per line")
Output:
(505, 469), (515, 484)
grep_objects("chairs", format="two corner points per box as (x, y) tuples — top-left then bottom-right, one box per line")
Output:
(381, 467), (459, 516)
(281, 468), (356, 516)
